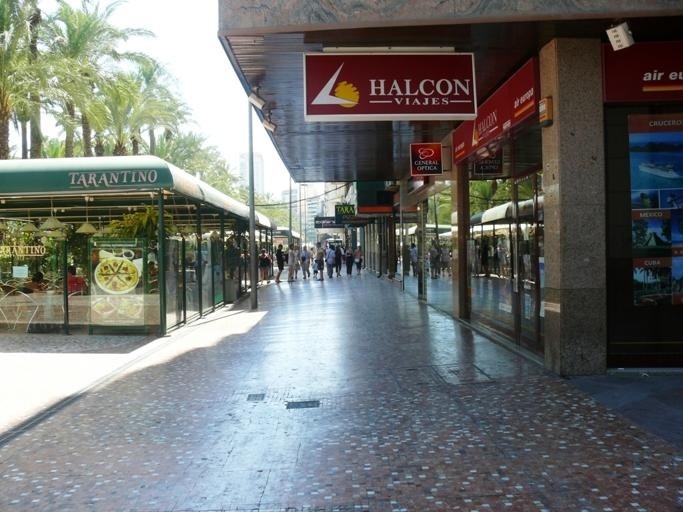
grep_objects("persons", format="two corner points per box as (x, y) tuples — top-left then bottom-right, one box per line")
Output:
(407, 235), (500, 279)
(148, 261), (158, 286)
(25, 272), (48, 291)
(241, 242), (364, 285)
(59, 266), (88, 296)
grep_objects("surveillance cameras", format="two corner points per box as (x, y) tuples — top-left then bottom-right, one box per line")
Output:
(262, 119), (277, 133)
(248, 93), (266, 110)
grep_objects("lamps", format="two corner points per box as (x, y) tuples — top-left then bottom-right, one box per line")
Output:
(0, 196), (97, 237)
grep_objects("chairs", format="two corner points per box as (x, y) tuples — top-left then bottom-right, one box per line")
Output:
(0, 275), (88, 333)
